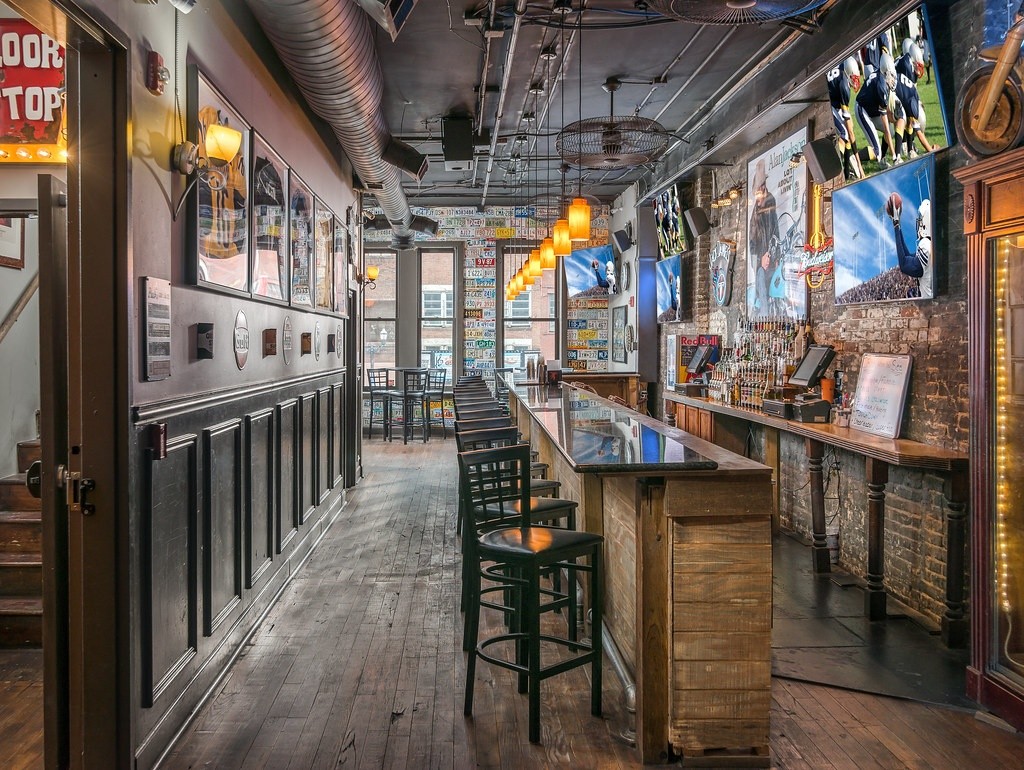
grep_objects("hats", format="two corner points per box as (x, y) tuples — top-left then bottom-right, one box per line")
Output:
(752, 159), (769, 193)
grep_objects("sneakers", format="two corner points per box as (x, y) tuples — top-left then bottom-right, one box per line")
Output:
(897, 154), (905, 164)
(909, 150), (918, 158)
(878, 157), (892, 169)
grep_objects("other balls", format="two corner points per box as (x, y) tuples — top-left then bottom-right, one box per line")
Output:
(598, 450), (605, 457)
(592, 260), (599, 269)
(667, 272), (674, 281)
(886, 194), (903, 216)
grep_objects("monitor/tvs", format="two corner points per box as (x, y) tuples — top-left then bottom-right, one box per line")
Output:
(685, 344), (714, 375)
(788, 344), (836, 389)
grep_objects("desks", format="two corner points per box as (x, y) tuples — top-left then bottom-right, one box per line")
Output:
(662, 391), (970, 649)
(379, 367), (427, 441)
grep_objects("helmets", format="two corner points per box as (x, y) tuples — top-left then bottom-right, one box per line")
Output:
(902, 38), (914, 55)
(909, 44), (924, 63)
(880, 54), (897, 78)
(675, 276), (680, 300)
(918, 199), (932, 239)
(611, 439), (621, 456)
(605, 261), (614, 275)
(844, 56), (860, 79)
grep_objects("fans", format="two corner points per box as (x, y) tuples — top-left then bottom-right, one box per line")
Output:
(643, 0), (829, 26)
(553, 78), (669, 170)
(524, 166), (602, 223)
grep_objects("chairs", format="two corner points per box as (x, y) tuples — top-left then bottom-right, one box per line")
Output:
(366, 368), (606, 745)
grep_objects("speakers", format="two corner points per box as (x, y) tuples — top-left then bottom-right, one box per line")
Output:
(800, 137), (842, 185)
(684, 207), (710, 238)
(611, 230), (632, 253)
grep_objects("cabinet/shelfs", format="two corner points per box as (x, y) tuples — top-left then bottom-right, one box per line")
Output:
(686, 405), (712, 442)
(676, 403), (686, 431)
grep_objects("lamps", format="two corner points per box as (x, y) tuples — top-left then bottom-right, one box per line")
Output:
(505, 174), (516, 301)
(539, 58), (556, 271)
(684, 207), (719, 238)
(553, 10), (572, 257)
(528, 92), (543, 278)
(172, 123), (242, 222)
(568, 0), (591, 242)
(520, 122), (535, 287)
(356, 265), (380, 294)
(611, 230), (636, 254)
(788, 152), (803, 168)
(134, 0), (197, 14)
(514, 143), (527, 293)
(800, 134), (842, 185)
(709, 186), (742, 209)
(509, 161), (520, 297)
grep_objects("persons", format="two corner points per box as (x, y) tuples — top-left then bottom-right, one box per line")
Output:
(823, 9), (939, 179)
(884, 192), (932, 301)
(748, 161), (784, 321)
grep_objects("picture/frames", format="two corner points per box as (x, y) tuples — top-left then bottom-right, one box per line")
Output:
(186, 63), (251, 301)
(289, 168), (316, 313)
(251, 126), (290, 308)
(612, 305), (628, 364)
(315, 194), (350, 320)
